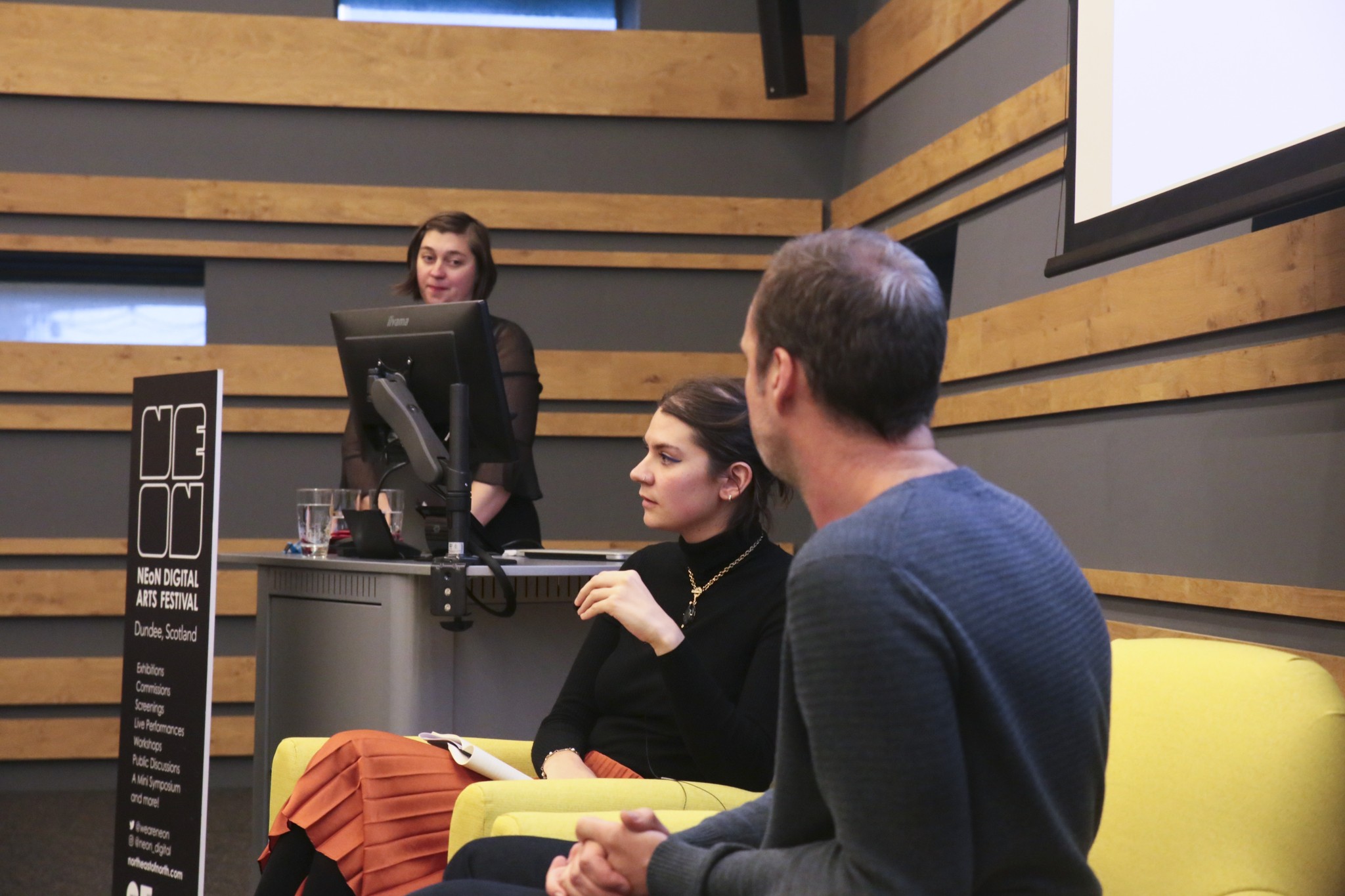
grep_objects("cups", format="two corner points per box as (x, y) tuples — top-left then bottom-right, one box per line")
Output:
(369, 488), (404, 537)
(297, 489), (333, 558)
(331, 488), (362, 531)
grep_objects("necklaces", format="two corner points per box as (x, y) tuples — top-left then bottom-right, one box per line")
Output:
(680, 533), (764, 628)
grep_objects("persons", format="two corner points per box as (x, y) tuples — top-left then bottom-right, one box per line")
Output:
(341, 210), (542, 553)
(405, 228), (1110, 896)
(254, 376), (795, 896)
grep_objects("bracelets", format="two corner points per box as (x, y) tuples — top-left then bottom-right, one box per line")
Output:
(540, 748), (578, 779)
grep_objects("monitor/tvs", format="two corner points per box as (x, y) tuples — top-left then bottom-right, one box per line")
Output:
(329, 299), (516, 464)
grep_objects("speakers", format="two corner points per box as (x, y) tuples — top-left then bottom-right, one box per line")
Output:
(755, 1), (809, 100)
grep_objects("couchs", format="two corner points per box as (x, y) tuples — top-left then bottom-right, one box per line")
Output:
(268, 638), (1345, 896)
